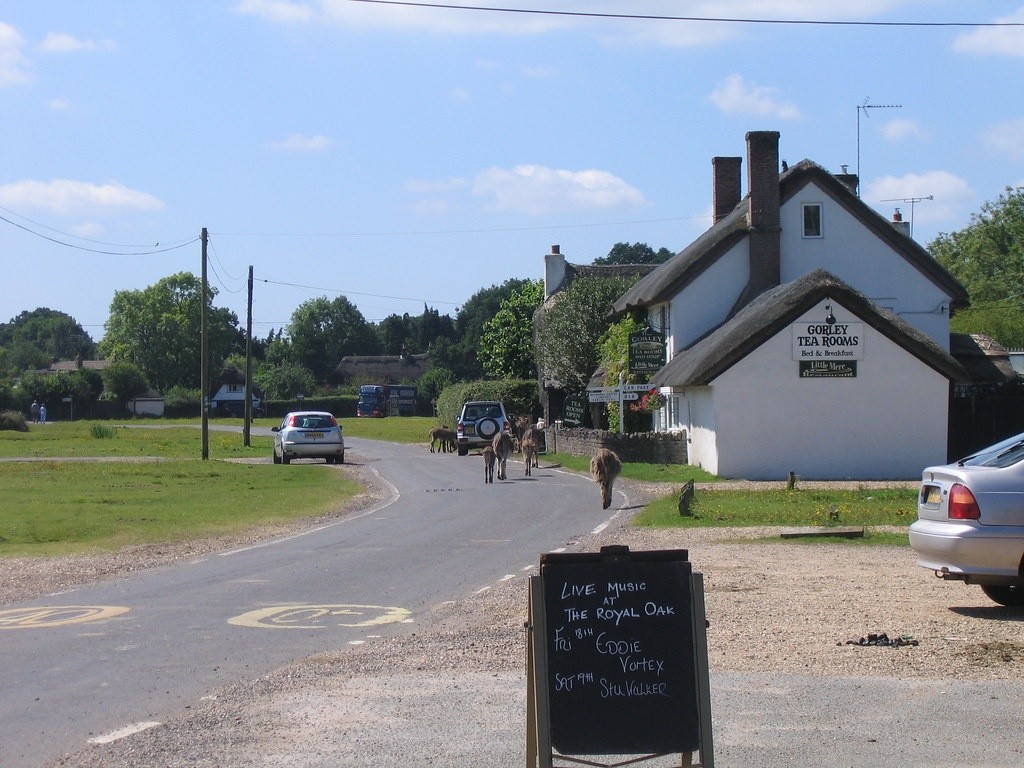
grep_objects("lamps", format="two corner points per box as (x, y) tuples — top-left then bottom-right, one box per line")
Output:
(825, 303), (836, 324)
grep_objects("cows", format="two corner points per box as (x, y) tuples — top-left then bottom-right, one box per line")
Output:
(427, 417), (539, 483)
(590, 446), (622, 510)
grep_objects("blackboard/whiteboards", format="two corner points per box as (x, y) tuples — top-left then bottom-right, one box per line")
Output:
(539, 550), (701, 757)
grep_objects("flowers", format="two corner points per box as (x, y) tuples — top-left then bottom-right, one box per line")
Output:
(630, 388), (668, 413)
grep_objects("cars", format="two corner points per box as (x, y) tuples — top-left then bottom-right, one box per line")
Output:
(908, 432), (1024, 607)
(270, 411), (346, 465)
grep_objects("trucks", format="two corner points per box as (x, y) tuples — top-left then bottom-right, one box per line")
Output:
(355, 384), (419, 418)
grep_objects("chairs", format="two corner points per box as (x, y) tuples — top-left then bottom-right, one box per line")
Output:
(317, 419), (328, 427)
(296, 418), (303, 427)
(468, 409), (478, 417)
(491, 408), (499, 417)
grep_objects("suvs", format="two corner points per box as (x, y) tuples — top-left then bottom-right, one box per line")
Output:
(455, 400), (513, 456)
